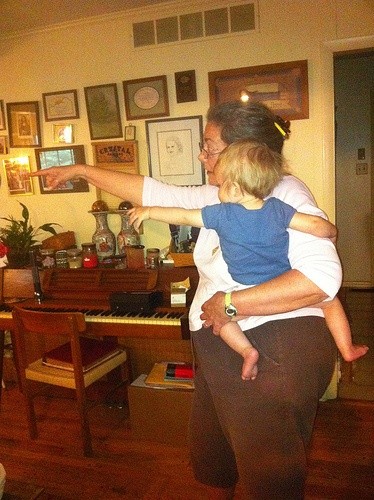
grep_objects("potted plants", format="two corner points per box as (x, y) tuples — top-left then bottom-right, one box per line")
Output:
(0, 200), (64, 267)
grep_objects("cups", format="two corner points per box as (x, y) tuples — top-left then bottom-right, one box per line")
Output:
(41, 249), (55, 269)
(56, 250), (68, 268)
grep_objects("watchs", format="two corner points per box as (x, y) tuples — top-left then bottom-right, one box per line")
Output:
(224, 291), (238, 319)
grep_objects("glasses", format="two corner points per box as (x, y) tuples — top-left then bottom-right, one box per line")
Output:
(199, 142), (226, 160)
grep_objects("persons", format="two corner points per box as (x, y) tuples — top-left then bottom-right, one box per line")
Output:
(26, 100), (343, 500)
(19, 115), (31, 136)
(125, 139), (369, 382)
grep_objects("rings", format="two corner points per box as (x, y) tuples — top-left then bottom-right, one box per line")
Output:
(204, 322), (210, 327)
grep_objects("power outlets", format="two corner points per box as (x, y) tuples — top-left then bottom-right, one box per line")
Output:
(356, 163), (369, 176)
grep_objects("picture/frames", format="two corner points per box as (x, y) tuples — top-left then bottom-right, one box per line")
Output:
(3, 157), (34, 198)
(83, 83), (124, 142)
(42, 90), (80, 123)
(0, 101), (10, 155)
(6, 101), (43, 148)
(207, 60), (310, 121)
(142, 116), (206, 187)
(122, 75), (170, 122)
(34, 145), (90, 196)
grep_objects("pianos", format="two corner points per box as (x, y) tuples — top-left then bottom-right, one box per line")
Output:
(9, 256), (201, 403)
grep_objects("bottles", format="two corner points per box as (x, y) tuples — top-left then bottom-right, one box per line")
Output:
(115, 209), (141, 255)
(66, 249), (82, 269)
(146, 248), (159, 269)
(88, 210), (116, 263)
(81, 243), (98, 268)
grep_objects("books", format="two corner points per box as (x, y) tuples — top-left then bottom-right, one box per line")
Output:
(144, 360), (195, 389)
(41, 335), (123, 373)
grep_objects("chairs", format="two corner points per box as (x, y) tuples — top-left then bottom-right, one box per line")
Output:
(10, 305), (134, 456)
